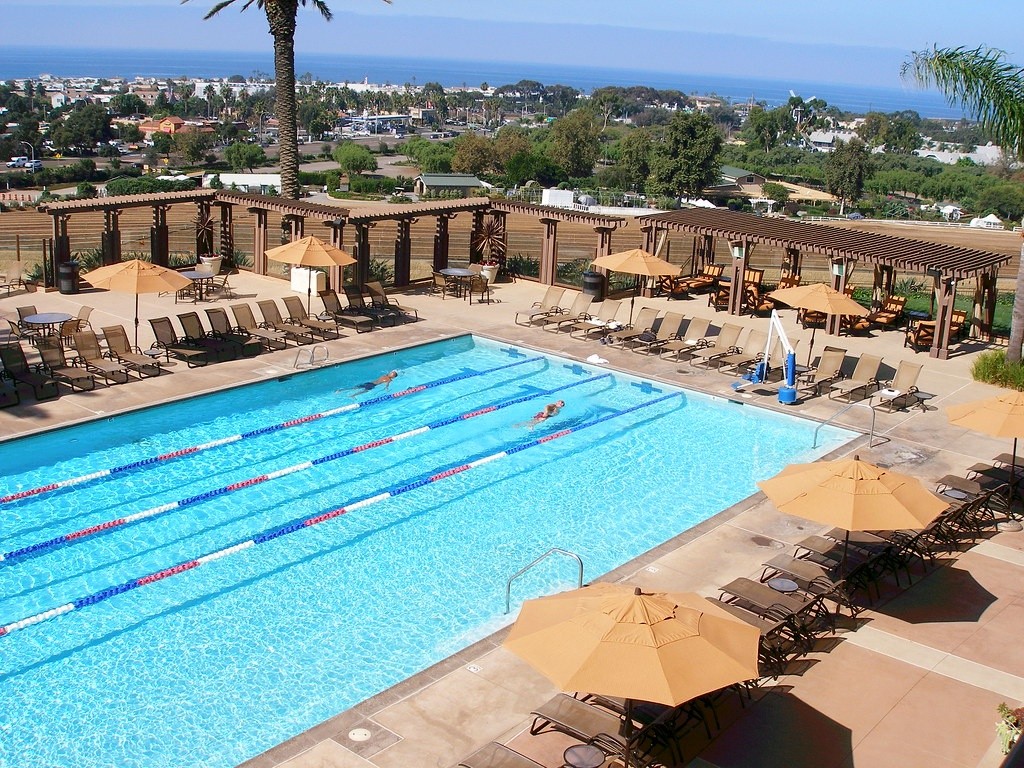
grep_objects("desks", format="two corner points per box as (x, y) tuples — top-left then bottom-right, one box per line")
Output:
(143, 349), (164, 367)
(911, 392), (932, 413)
(795, 365), (810, 385)
(440, 268), (477, 298)
(317, 316), (333, 333)
(24, 312), (73, 350)
(181, 270), (216, 301)
(563, 744), (606, 768)
(768, 577), (799, 594)
(944, 489), (968, 501)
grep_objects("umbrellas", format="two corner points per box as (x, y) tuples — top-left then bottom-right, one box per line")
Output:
(946, 386), (1024, 523)
(766, 281), (871, 367)
(757, 454), (951, 621)
(500, 580), (761, 768)
(263, 234), (357, 319)
(586, 247), (683, 330)
(77, 259), (193, 356)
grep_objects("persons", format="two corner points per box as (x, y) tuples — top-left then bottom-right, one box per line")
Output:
(375, 371), (398, 386)
(535, 400), (565, 422)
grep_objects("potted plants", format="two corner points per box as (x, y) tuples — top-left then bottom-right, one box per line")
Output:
(191, 212), (224, 275)
(469, 214), (507, 284)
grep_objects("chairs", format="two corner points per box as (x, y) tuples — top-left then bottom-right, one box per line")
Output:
(0, 260), (968, 410)
(454, 452), (1024, 768)
